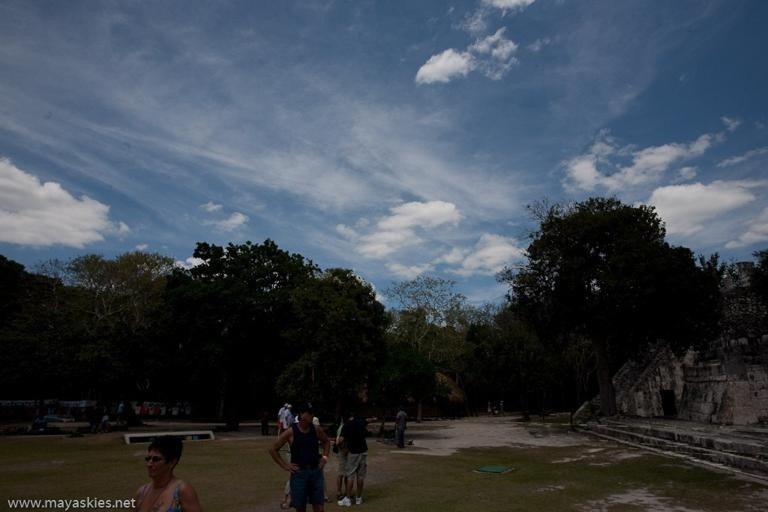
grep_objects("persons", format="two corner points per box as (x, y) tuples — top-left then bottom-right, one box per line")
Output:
(268, 404), (330, 512)
(131, 435), (203, 512)
(261, 401), (410, 509)
(31, 399), (192, 433)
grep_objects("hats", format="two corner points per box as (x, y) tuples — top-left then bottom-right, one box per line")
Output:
(299, 401), (316, 412)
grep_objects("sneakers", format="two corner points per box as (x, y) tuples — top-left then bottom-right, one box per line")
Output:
(280, 495), (364, 510)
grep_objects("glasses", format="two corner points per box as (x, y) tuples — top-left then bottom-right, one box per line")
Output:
(144, 454), (163, 462)
